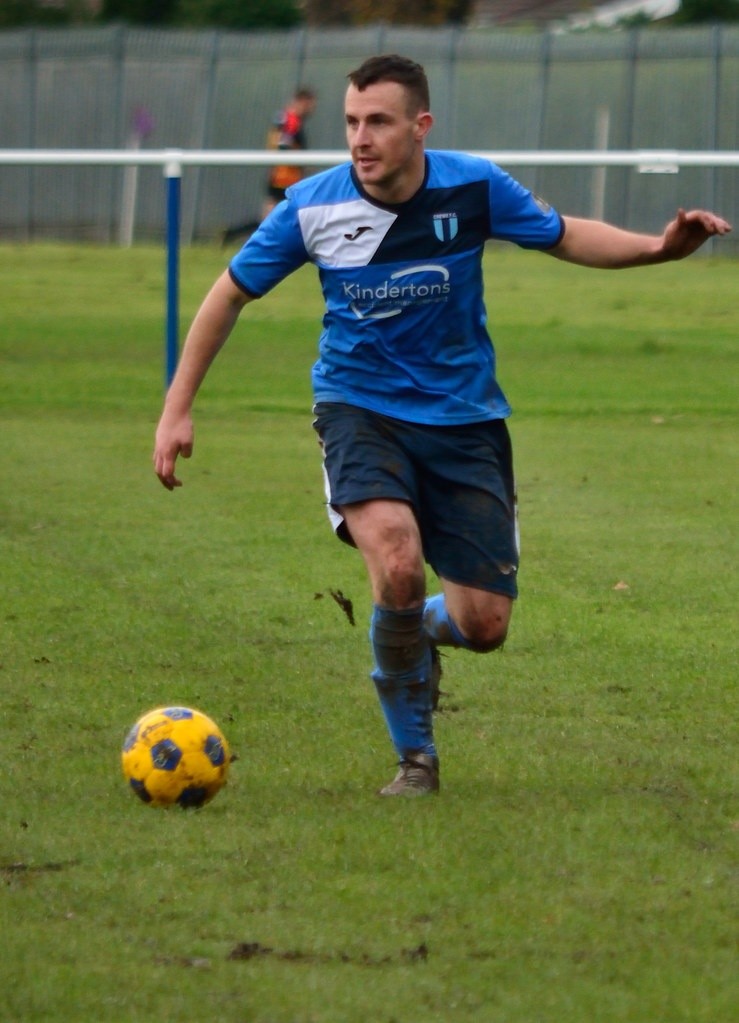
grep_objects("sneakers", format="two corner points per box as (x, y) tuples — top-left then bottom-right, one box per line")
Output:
(422, 642), (446, 701)
(378, 755), (444, 802)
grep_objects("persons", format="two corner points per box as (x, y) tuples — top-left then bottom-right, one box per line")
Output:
(214, 84), (323, 248)
(148, 55), (734, 802)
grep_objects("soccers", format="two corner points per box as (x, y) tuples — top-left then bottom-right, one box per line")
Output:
(121, 707), (233, 812)
